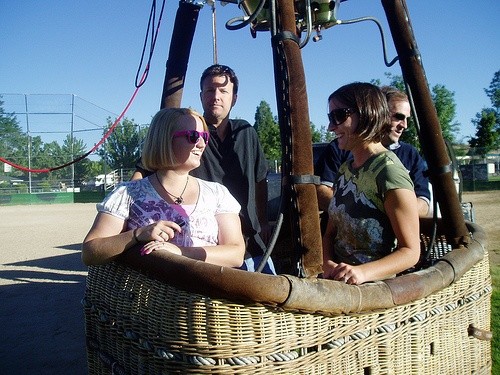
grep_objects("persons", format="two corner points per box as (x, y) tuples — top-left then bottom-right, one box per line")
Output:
(314, 86), (430, 234)
(81, 107), (245, 267)
(322, 83), (420, 285)
(130, 64), (276, 274)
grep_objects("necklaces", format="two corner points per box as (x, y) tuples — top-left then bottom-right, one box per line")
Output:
(156, 171), (189, 203)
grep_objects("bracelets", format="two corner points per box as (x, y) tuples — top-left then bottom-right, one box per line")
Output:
(133, 229), (139, 242)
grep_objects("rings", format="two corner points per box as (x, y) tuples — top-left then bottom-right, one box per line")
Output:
(159, 232), (162, 236)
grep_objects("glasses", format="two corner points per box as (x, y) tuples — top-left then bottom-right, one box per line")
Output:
(172, 130), (209, 147)
(327, 107), (355, 126)
(395, 112), (410, 124)
